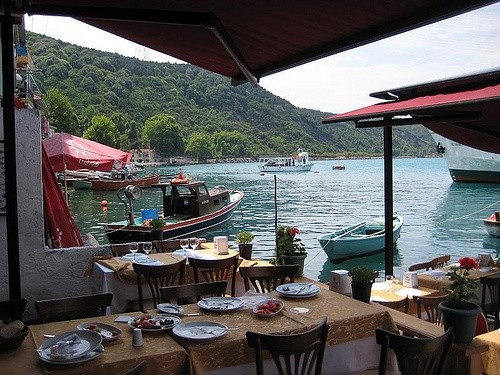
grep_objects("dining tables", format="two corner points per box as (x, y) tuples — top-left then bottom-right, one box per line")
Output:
(0, 311), (193, 375)
(145, 284), (401, 375)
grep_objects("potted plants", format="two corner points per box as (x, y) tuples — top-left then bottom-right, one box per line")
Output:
(149, 219), (166, 240)
(236, 231), (254, 260)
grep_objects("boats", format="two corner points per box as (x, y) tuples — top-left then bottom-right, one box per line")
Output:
(56, 158), (205, 192)
(97, 168), (245, 245)
(425, 128), (500, 184)
(316, 210), (403, 264)
(482, 210), (500, 238)
(258, 152), (317, 172)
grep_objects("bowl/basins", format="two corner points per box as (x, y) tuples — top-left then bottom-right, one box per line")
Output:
(0, 325), (30, 352)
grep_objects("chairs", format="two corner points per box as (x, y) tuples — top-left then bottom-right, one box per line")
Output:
(0, 238), (500, 375)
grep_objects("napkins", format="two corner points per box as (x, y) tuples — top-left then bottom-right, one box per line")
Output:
(113, 316), (136, 322)
(237, 291), (275, 310)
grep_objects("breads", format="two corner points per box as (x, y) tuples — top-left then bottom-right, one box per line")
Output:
(0, 320), (25, 338)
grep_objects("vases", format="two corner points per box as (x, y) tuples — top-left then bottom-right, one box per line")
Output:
(281, 254), (307, 278)
(437, 300), (481, 344)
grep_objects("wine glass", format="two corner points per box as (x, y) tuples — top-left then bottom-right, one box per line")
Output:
(129, 242), (139, 262)
(385, 274), (400, 292)
(143, 242), (152, 263)
(190, 238), (199, 258)
(180, 239), (189, 257)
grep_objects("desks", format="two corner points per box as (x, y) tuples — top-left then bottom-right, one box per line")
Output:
(89, 248), (257, 315)
(370, 282), (440, 319)
(416, 263), (500, 291)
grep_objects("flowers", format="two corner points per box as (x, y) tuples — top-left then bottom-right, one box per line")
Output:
(445, 257), (480, 308)
(269, 225), (308, 265)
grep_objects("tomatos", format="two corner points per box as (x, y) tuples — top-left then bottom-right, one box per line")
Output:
(257, 300), (281, 314)
(139, 316), (156, 327)
(90, 324), (96, 330)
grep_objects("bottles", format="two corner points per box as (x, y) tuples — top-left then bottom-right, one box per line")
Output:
(132, 329), (143, 347)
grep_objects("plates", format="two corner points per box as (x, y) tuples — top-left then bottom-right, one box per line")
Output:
(197, 297), (244, 311)
(276, 283), (320, 298)
(37, 329), (102, 362)
(127, 314), (181, 331)
(157, 303), (183, 313)
(38, 344), (104, 365)
(76, 321), (122, 341)
(172, 321), (228, 341)
(253, 299), (284, 318)
